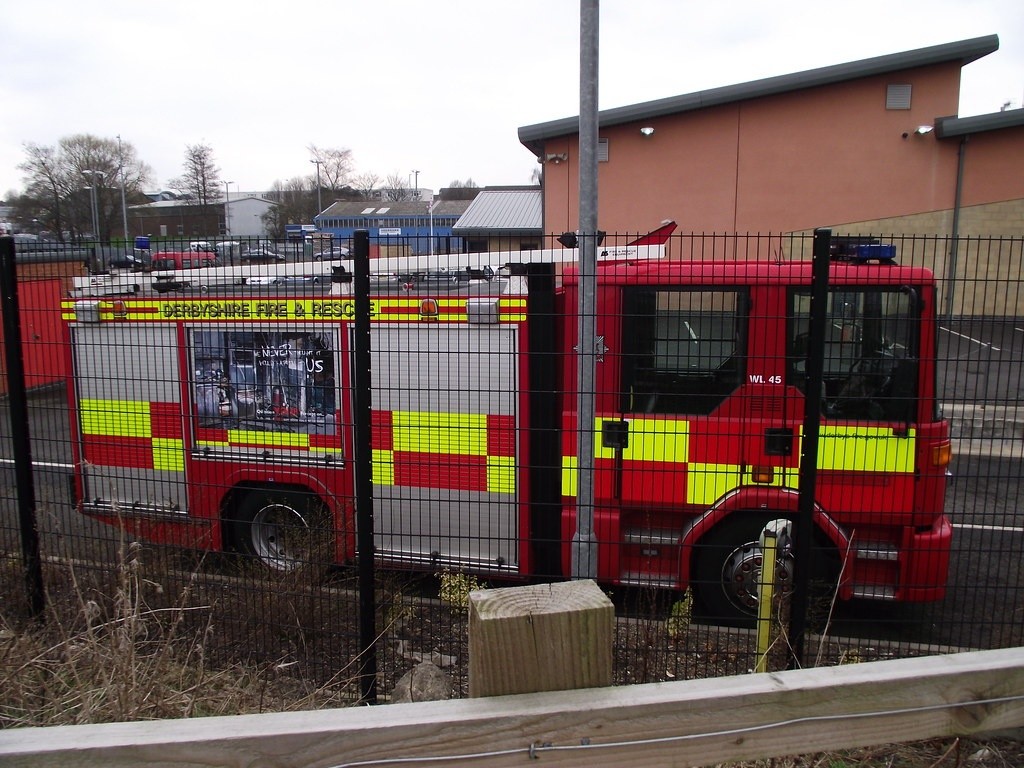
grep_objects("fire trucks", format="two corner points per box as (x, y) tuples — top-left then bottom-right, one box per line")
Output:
(59, 238), (952, 617)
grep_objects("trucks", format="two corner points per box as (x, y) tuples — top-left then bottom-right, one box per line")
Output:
(283, 224), (321, 243)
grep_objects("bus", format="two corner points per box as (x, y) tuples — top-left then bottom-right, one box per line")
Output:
(151, 250), (222, 290)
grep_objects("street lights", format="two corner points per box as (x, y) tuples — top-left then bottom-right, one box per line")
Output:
(81, 169), (106, 273)
(411, 168), (420, 200)
(32, 218), (65, 243)
(219, 180), (234, 235)
(310, 159), (327, 214)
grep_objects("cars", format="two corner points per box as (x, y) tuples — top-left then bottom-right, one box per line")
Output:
(259, 239), (271, 247)
(108, 254), (147, 268)
(216, 240), (240, 248)
(242, 248), (286, 264)
(0, 214), (93, 251)
(312, 246), (349, 261)
(189, 241), (211, 251)
